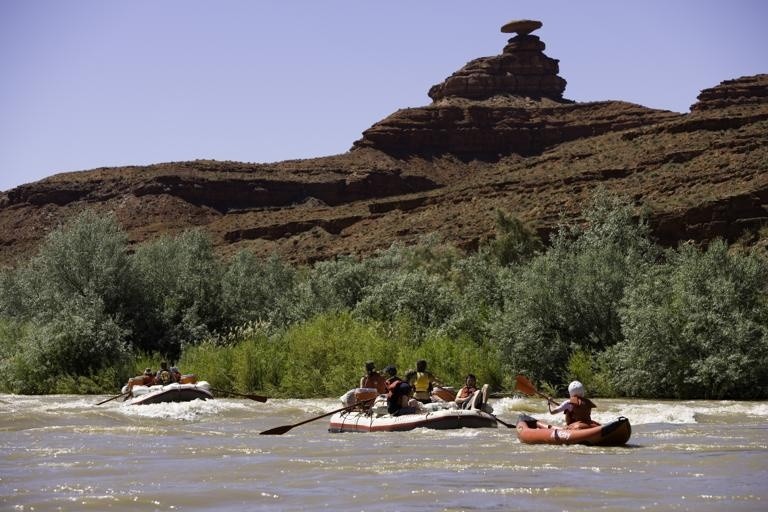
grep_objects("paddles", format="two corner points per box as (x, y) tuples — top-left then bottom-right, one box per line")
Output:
(210, 388), (266, 403)
(434, 383), (516, 427)
(259, 398), (377, 435)
(514, 375), (562, 407)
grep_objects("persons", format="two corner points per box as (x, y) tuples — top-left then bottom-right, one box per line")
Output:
(359, 360), (386, 395)
(387, 368), (422, 416)
(411, 357), (435, 404)
(548, 379), (597, 429)
(454, 374), (490, 412)
(140, 359), (181, 386)
(383, 365), (402, 385)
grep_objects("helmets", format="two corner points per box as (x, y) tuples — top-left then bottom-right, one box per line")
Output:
(568, 381), (584, 398)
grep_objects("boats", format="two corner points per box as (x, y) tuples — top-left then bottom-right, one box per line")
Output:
(122, 379), (214, 405)
(515, 411), (633, 447)
(327, 406), (500, 433)
